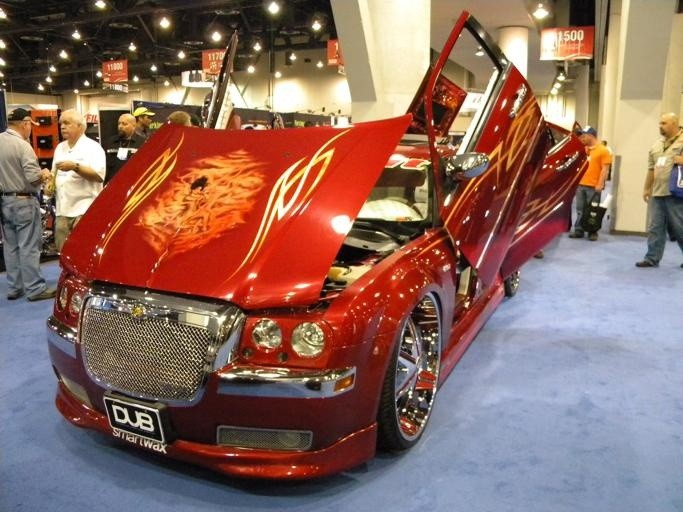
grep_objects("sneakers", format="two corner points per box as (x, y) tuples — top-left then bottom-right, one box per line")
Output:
(588, 233), (598, 241)
(569, 231), (584, 237)
(636, 260), (653, 267)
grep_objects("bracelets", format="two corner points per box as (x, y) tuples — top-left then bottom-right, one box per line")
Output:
(72, 160), (79, 175)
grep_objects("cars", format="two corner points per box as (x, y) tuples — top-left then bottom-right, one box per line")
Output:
(47, 10), (589, 482)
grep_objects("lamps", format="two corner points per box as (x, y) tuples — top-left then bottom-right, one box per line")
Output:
(533, 3), (549, 19)
(554, 72), (565, 89)
(475, 47), (484, 57)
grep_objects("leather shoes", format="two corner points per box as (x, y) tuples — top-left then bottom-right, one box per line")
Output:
(28, 288), (57, 300)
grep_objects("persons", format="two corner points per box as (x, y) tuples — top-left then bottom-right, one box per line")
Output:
(570, 128), (585, 145)
(168, 111), (191, 127)
(133, 105), (157, 140)
(43, 108), (106, 253)
(103, 113), (146, 187)
(568, 125), (611, 240)
(1, 107), (58, 301)
(636, 110), (683, 270)
(600, 140), (611, 180)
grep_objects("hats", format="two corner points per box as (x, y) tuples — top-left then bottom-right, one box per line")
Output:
(8, 108), (40, 126)
(134, 107), (155, 117)
(576, 125), (597, 137)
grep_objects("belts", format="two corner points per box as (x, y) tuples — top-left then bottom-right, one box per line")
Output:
(1, 191), (36, 196)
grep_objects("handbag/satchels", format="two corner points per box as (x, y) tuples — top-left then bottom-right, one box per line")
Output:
(580, 204), (607, 231)
(670, 163), (683, 197)
